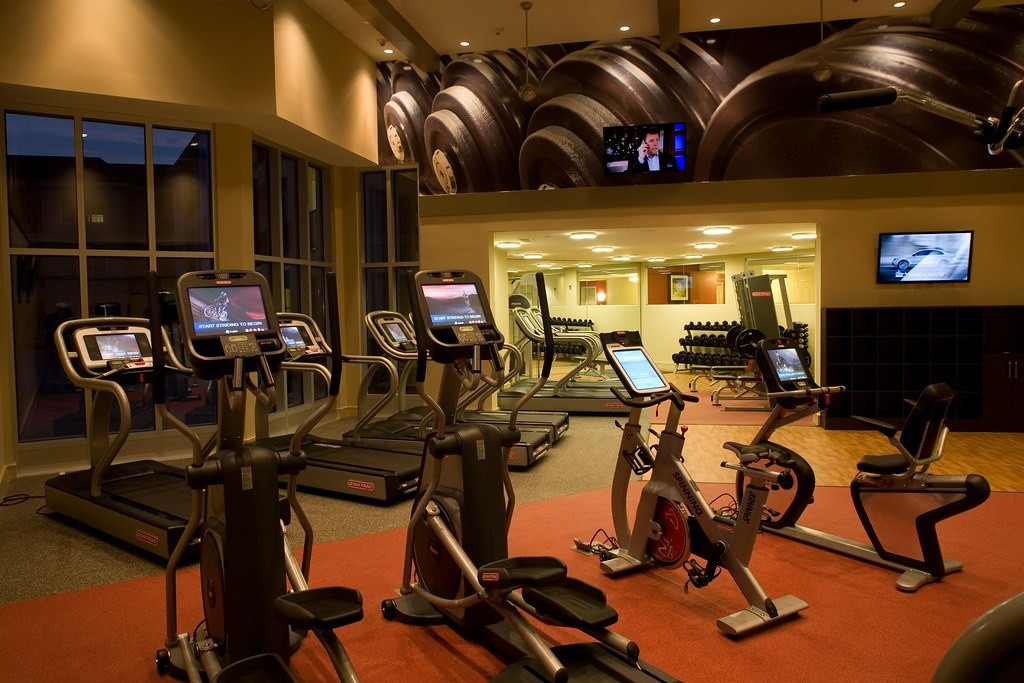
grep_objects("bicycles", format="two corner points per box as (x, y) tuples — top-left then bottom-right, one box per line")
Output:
(204, 300), (228, 322)
(736, 386), (991, 591)
(600, 382), (811, 638)
(104, 343), (118, 354)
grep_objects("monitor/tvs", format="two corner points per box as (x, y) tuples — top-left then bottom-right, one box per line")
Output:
(377, 317), (417, 348)
(177, 270), (277, 340)
(414, 268), (494, 330)
(278, 320), (320, 350)
(74, 324), (155, 369)
(876, 230), (974, 284)
(602, 122), (688, 178)
(767, 347), (808, 381)
(607, 345), (670, 394)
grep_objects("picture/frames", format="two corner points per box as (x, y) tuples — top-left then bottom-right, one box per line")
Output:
(668, 272), (690, 304)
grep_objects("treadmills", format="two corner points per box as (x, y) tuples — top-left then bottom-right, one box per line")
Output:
(45, 306), (641, 562)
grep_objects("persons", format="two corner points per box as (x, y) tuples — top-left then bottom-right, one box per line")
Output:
(632, 131), (668, 172)
(213, 292), (229, 317)
(42, 303), (68, 395)
(462, 290), (470, 309)
(775, 351), (787, 369)
(111, 337), (118, 347)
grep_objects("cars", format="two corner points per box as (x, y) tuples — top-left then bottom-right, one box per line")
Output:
(893, 249), (961, 270)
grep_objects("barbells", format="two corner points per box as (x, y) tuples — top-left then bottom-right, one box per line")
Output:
(672, 321), (812, 370)
(531, 316), (594, 355)
